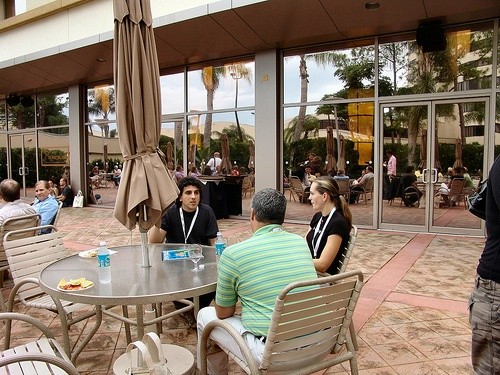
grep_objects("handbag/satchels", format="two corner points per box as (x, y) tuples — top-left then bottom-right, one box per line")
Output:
(465, 176), (488, 222)
(72, 190), (84, 208)
(124, 331), (176, 375)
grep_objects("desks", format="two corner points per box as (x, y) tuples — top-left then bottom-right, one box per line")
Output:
(414, 179), (449, 208)
(221, 173), (244, 217)
(38, 239), (225, 369)
(100, 171), (114, 188)
(196, 174), (230, 221)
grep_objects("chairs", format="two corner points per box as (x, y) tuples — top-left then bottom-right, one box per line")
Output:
(335, 177), (350, 205)
(49, 199), (67, 234)
(243, 177), (255, 199)
(3, 223), (130, 355)
(288, 175), (307, 205)
(313, 223), (358, 287)
(0, 213), (43, 272)
(436, 177), (468, 209)
(199, 268), (365, 375)
(89, 176), (99, 190)
(0, 311), (82, 375)
(351, 176), (374, 205)
(390, 176), (419, 208)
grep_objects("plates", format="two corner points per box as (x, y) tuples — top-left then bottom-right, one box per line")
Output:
(57, 282), (94, 292)
(79, 251), (110, 258)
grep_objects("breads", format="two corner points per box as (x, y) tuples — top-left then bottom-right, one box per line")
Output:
(90, 251), (98, 257)
(59, 277), (93, 290)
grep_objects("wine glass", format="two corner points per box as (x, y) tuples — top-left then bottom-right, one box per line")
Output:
(188, 243), (202, 272)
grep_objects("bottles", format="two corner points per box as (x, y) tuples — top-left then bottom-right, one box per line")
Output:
(97, 241), (111, 283)
(215, 231), (226, 264)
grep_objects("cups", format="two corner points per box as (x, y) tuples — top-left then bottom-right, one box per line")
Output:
(216, 238), (228, 248)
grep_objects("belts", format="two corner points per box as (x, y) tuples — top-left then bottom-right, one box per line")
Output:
(255, 335), (267, 344)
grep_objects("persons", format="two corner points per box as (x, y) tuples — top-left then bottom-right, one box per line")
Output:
(294, 152), (338, 204)
(401, 166), (423, 207)
(306, 175), (352, 288)
(468, 154), (500, 375)
(231, 165), (239, 175)
(30, 180), (59, 234)
(439, 167), (476, 207)
(113, 166), (122, 188)
(205, 152), (222, 176)
(175, 165), (183, 182)
(56, 178), (74, 207)
(0, 179), (36, 226)
(197, 188), (331, 375)
(189, 167), (202, 178)
(383, 150), (396, 199)
(89, 166), (104, 189)
(148, 178), (220, 329)
(48, 181), (58, 197)
(333, 169), (352, 188)
(346, 166), (374, 204)
(239, 167), (247, 174)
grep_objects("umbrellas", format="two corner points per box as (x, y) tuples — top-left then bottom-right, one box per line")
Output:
(220, 132), (232, 176)
(452, 137), (463, 170)
(248, 139), (256, 169)
(166, 141), (175, 170)
(325, 125), (346, 175)
(114, 1), (180, 268)
(419, 128), (441, 169)
